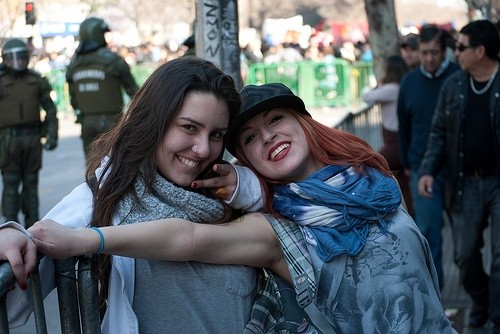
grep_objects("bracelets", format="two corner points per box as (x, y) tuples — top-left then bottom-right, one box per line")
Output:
(92, 227), (104, 254)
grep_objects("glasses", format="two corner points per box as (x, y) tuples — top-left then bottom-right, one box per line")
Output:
(455, 43), (469, 52)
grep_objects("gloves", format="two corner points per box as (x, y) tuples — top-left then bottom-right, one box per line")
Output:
(43, 119), (58, 149)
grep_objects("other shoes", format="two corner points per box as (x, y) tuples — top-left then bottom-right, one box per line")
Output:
(470, 305), (492, 327)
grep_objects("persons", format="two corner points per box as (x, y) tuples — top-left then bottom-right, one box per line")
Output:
(363, 20), (500, 334)
(0, 37), (58, 230)
(176, 19), (197, 57)
(0, 55), (264, 334)
(25, 83), (460, 334)
(240, 27), (374, 99)
(65, 18), (140, 162)
(26, 39), (168, 76)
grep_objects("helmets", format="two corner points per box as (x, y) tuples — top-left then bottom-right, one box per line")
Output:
(80, 18), (110, 44)
(2, 39), (29, 72)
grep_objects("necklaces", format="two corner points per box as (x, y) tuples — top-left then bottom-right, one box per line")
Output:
(471, 62), (498, 94)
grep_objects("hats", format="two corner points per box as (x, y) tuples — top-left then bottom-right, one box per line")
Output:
(225, 81), (305, 158)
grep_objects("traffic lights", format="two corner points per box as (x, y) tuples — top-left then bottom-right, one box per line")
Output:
(25, 3), (35, 24)
